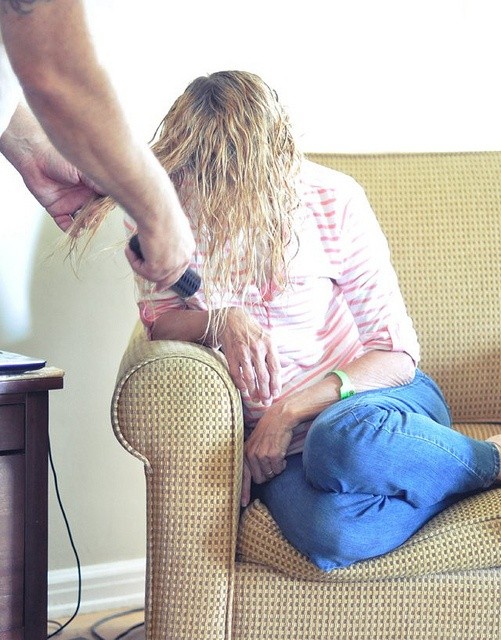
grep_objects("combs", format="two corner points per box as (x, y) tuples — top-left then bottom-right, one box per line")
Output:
(130, 235), (201, 297)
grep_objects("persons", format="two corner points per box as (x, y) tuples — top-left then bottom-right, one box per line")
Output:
(0, 0), (198, 294)
(52, 69), (501, 572)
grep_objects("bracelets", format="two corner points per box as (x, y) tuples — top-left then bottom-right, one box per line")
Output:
(325, 369), (356, 399)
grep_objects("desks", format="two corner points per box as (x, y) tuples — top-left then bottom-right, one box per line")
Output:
(0, 350), (68, 640)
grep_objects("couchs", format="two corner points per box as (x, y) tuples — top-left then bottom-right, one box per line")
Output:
(108, 146), (501, 638)
